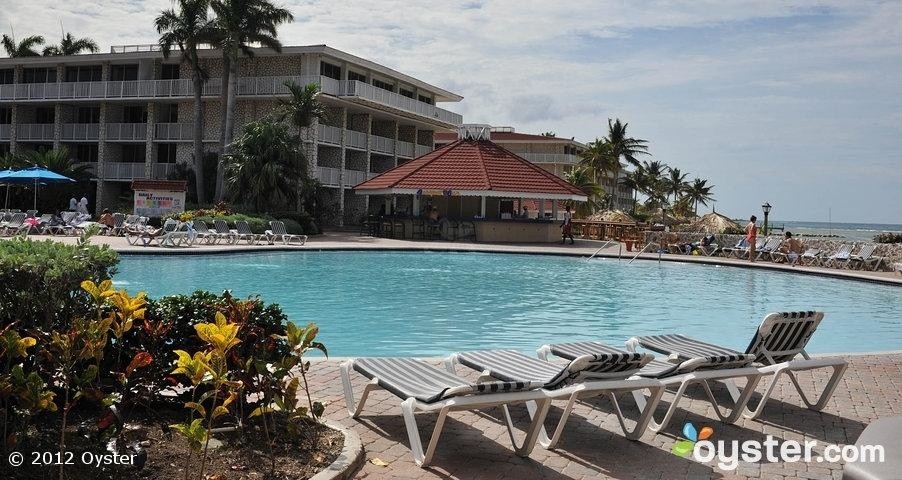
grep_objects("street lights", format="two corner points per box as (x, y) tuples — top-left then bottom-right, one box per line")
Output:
(761, 202), (773, 236)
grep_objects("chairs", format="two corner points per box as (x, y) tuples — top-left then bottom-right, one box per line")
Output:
(338, 347), (540, 469)
(358, 213), (474, 240)
(1, 209), (154, 236)
(445, 343), (666, 449)
(629, 309), (848, 421)
(124, 215), (307, 246)
(537, 340), (761, 438)
(650, 233), (886, 271)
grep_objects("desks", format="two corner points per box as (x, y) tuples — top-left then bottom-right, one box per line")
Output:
(620, 239), (638, 252)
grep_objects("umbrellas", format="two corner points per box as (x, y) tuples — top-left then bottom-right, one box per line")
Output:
(0, 163), (77, 210)
(0, 167), (20, 210)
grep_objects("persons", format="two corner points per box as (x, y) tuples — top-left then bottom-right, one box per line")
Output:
(429, 207), (439, 222)
(522, 206), (529, 218)
(779, 230), (800, 254)
(352, 209), (361, 224)
(743, 215), (758, 262)
(96, 208), (115, 236)
(473, 211), (481, 218)
(560, 205), (576, 245)
(679, 236), (708, 253)
(76, 198), (81, 212)
(774, 232), (788, 264)
(68, 194), (77, 211)
(81, 192), (90, 214)
(513, 210), (518, 217)
